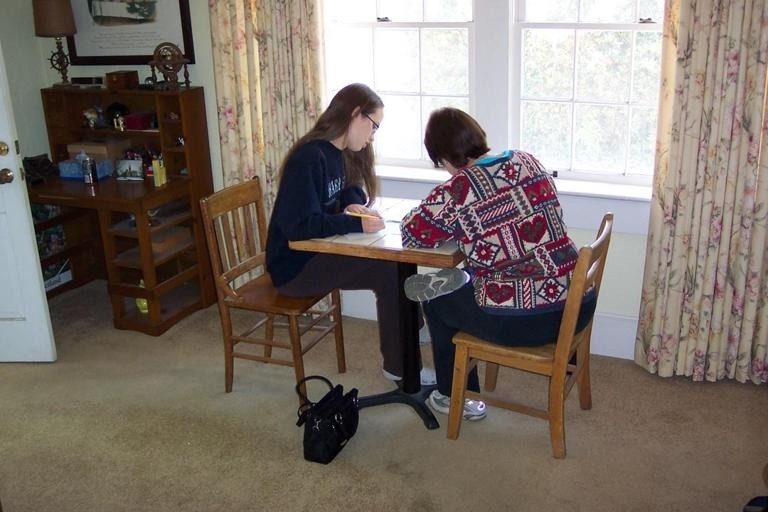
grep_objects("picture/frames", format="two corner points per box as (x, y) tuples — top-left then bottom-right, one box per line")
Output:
(66, 0), (196, 66)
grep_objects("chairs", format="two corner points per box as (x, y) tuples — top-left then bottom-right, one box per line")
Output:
(198, 175), (347, 408)
(447, 211), (613, 460)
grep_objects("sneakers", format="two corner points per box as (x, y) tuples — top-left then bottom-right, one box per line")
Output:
(429, 389), (488, 421)
(403, 265), (471, 302)
(379, 363), (437, 386)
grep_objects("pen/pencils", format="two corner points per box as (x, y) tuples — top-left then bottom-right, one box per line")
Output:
(345, 212), (383, 219)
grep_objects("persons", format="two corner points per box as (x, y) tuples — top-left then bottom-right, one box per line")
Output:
(263, 82), (437, 388)
(399, 104), (597, 423)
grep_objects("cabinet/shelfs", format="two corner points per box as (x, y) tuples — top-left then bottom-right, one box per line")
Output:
(39, 86), (214, 182)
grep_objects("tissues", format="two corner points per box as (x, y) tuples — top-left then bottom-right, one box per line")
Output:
(59, 149), (112, 178)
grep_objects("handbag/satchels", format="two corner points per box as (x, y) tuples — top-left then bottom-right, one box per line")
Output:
(293, 374), (360, 464)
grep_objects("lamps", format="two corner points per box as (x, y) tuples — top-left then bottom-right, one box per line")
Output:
(33, 0), (77, 88)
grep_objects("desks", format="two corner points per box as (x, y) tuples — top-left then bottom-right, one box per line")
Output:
(290, 198), (466, 428)
(27, 174), (230, 337)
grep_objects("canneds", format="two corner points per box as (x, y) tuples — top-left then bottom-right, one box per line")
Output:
(82, 159), (97, 184)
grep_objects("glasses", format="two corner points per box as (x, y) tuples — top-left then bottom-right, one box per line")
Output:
(434, 158), (446, 169)
(361, 111), (380, 130)
